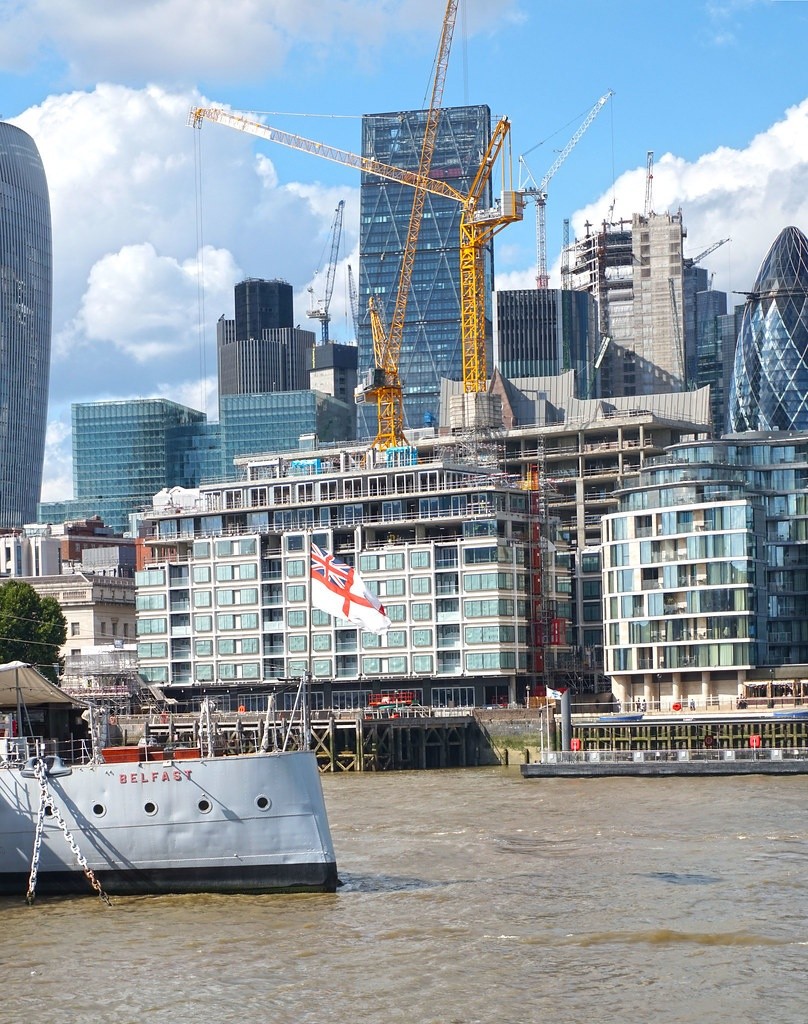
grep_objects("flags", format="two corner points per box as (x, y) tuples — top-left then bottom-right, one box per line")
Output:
(310, 542), (391, 635)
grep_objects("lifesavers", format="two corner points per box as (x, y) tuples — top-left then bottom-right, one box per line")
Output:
(672, 703), (681, 711)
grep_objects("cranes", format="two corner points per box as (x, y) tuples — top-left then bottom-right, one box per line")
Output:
(188, 0), (732, 472)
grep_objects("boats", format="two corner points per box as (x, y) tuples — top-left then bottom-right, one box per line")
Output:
(0, 526), (390, 905)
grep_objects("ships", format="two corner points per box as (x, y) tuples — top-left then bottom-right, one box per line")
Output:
(520, 685), (808, 776)
